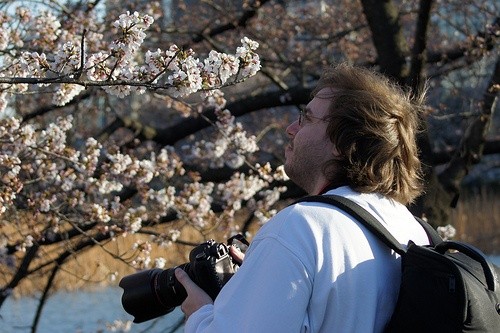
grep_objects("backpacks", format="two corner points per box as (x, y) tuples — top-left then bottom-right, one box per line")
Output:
(288, 195), (500, 333)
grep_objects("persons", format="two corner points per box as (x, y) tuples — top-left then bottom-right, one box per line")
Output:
(175, 61), (457, 332)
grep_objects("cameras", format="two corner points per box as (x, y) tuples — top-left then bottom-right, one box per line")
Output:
(118, 240), (239, 324)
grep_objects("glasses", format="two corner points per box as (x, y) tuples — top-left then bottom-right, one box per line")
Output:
(297, 108), (331, 126)
